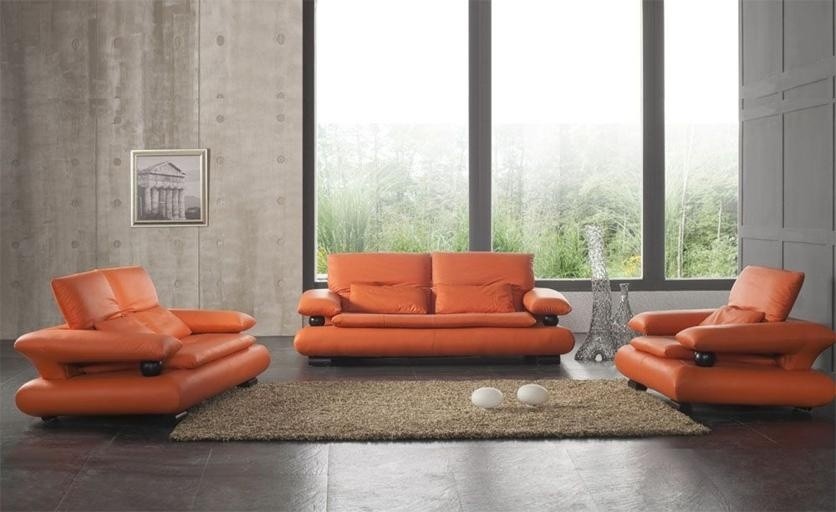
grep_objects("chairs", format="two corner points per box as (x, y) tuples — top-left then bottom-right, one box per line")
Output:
(615, 265), (836, 416)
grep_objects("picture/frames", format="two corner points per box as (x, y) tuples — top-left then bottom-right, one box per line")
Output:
(131, 148), (209, 228)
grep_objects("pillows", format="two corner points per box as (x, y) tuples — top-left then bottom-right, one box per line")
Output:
(327, 252), (536, 314)
(698, 265), (804, 326)
(51, 264), (193, 339)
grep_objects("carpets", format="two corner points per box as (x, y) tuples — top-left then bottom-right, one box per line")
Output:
(170, 379), (713, 442)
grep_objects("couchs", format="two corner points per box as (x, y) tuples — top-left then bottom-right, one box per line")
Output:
(294, 252), (575, 366)
(14, 265), (270, 427)
